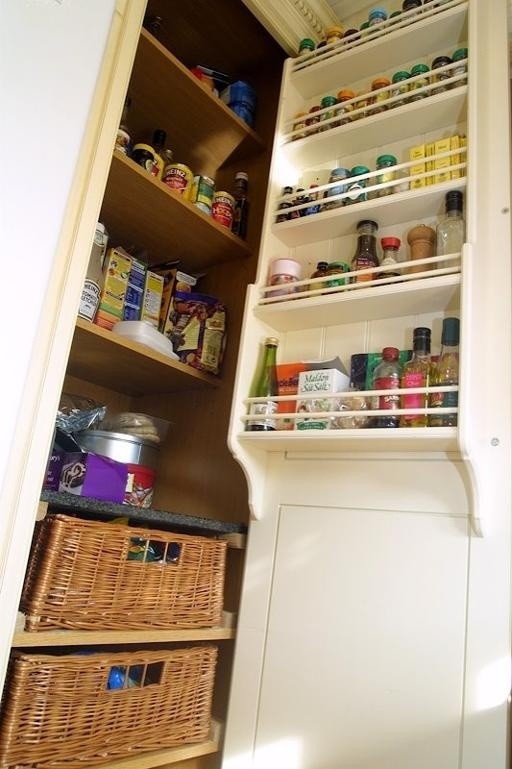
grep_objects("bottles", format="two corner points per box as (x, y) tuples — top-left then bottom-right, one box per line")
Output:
(276, 155), (400, 225)
(267, 189), (466, 307)
(276, 46), (469, 142)
(431, 318), (457, 426)
(246, 337), (280, 430)
(372, 348), (402, 427)
(115, 101), (259, 239)
(403, 327), (432, 427)
(71, 223), (110, 324)
(287, 0), (442, 60)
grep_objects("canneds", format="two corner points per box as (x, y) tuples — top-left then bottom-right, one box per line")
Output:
(293, 48), (468, 142)
(326, 262), (351, 294)
(299, 0), (441, 70)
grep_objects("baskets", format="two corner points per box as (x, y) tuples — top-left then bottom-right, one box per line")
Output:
(18, 513), (229, 633)
(0, 642), (219, 769)
